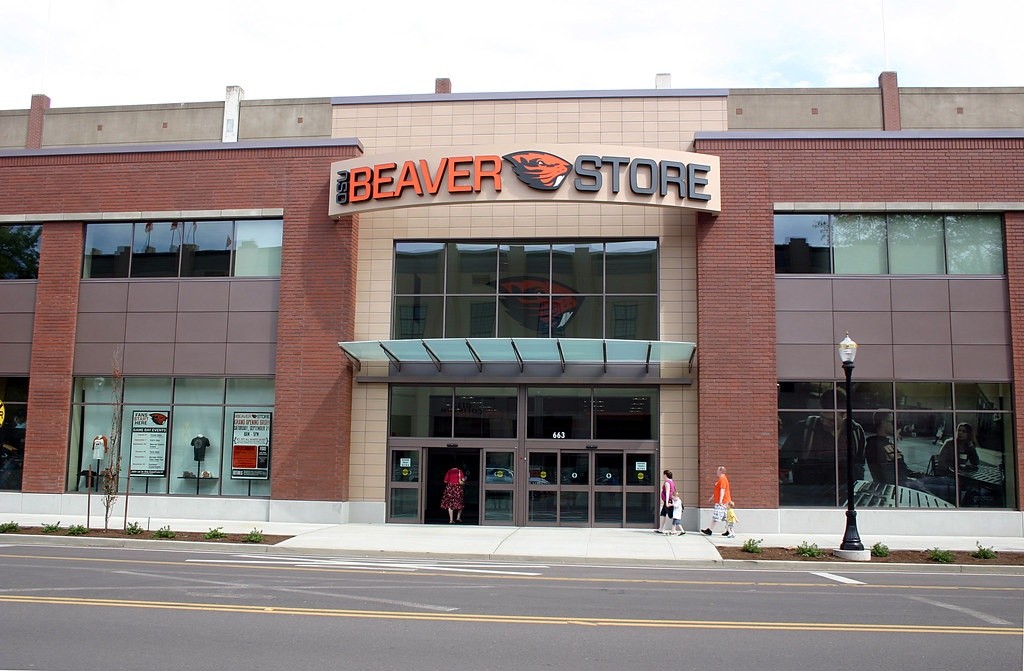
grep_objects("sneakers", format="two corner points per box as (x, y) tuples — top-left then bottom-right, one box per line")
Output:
(654, 530), (663, 534)
(722, 531), (729, 535)
(701, 528), (712, 536)
(667, 531), (677, 535)
(678, 531), (686, 536)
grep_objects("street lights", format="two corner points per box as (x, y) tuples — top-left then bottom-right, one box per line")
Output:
(838, 330), (865, 551)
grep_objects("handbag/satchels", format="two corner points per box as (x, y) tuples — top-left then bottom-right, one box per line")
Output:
(459, 469), (465, 485)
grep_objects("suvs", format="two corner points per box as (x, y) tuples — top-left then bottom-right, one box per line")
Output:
(486, 468), (550, 499)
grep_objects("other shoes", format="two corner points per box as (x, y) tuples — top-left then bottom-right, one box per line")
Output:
(449, 521), (456, 524)
(728, 532), (735, 538)
(456, 518), (463, 523)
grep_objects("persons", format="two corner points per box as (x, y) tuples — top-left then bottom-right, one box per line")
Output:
(91, 435), (109, 459)
(865, 408), (934, 496)
(440, 462), (466, 524)
(776, 387), (865, 485)
(190, 434), (210, 461)
(654, 470), (686, 536)
(700, 466), (739, 538)
(938, 423), (980, 502)
